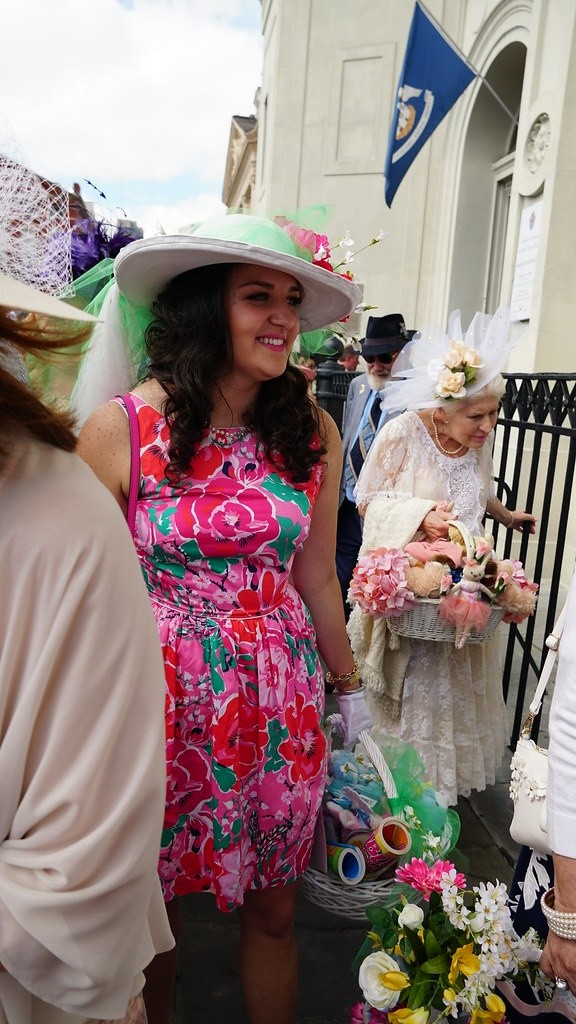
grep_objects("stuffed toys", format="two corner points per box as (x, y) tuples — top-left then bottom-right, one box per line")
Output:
(403, 524), (537, 649)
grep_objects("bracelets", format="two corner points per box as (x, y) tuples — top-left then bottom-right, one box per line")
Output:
(327, 663), (365, 694)
(541, 887), (576, 941)
(506, 511), (514, 527)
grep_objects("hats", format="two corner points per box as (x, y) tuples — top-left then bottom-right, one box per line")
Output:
(351, 313), (421, 356)
(0, 272), (105, 323)
(111, 213), (362, 334)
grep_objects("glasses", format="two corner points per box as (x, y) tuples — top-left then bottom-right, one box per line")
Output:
(362, 353), (399, 364)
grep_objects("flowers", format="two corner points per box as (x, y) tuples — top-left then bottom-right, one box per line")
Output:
(501, 557), (540, 625)
(340, 753), (386, 793)
(347, 804), (541, 1024)
(273, 213), (389, 354)
(347, 546), (418, 619)
(433, 339), (487, 405)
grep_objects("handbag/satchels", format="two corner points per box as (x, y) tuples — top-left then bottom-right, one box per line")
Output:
(507, 607), (567, 857)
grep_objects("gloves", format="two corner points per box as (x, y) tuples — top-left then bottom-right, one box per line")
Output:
(334, 689), (374, 753)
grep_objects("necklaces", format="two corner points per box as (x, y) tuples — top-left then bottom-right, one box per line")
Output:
(432, 409), (463, 454)
(205, 422), (244, 436)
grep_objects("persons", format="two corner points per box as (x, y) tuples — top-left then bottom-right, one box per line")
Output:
(74, 214), (370, 1024)
(0, 275), (179, 1023)
(344, 309), (537, 810)
(335, 312), (421, 624)
(339, 339), (361, 371)
(540, 581), (576, 988)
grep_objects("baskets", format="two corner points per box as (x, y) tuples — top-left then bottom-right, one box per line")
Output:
(293, 729), (453, 920)
(386, 519), (506, 643)
